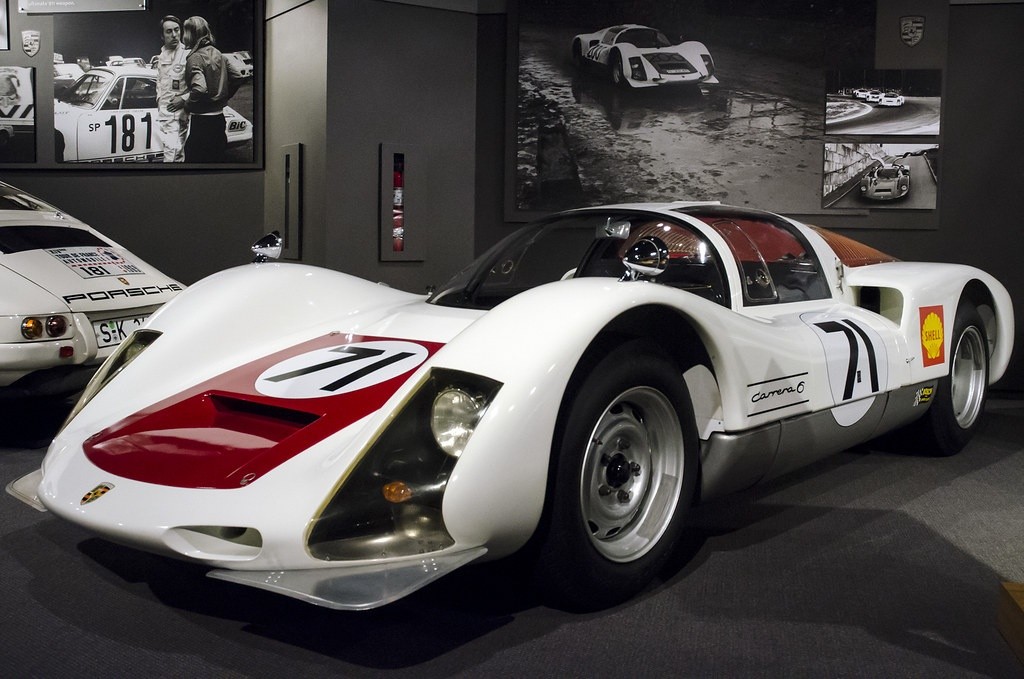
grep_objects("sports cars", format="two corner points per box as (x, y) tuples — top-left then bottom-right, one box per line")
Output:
(571, 24), (719, 96)
(5, 199), (1015, 622)
(859, 151), (913, 200)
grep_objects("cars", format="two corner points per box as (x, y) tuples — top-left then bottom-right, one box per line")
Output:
(221, 51), (253, 79)
(99, 56), (123, 66)
(878, 89), (905, 106)
(865, 90), (885, 103)
(109, 58), (146, 69)
(0, 179), (188, 389)
(54, 64), (85, 93)
(150, 55), (159, 69)
(54, 66), (253, 164)
(852, 89), (868, 99)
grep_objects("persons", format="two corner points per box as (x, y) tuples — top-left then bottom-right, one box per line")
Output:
(156, 15), (242, 163)
